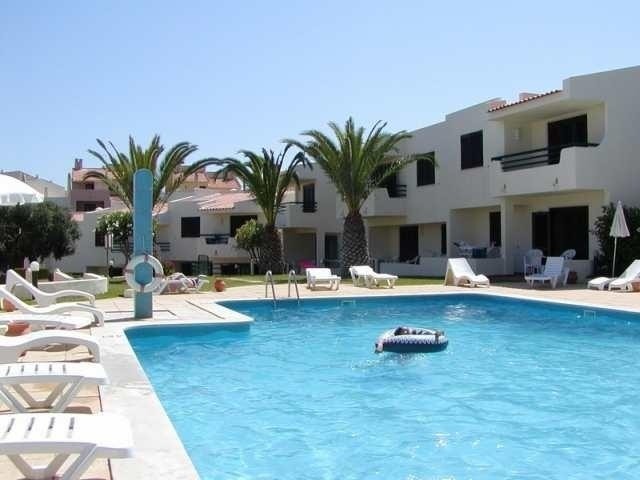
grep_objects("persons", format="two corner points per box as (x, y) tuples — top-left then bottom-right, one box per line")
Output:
(394, 326), (409, 335)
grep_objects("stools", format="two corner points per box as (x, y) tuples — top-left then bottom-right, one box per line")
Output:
(1, 355), (135, 479)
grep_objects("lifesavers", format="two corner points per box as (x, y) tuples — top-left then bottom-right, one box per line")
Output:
(123, 254), (164, 294)
(375, 326), (448, 352)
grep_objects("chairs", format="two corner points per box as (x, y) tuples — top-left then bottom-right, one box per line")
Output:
(443, 255), (490, 288)
(304, 265), (399, 291)
(1, 266), (107, 330)
(520, 248), (640, 292)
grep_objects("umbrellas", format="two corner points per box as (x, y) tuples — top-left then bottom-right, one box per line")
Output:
(609, 200), (630, 277)
(0, 174), (44, 206)
